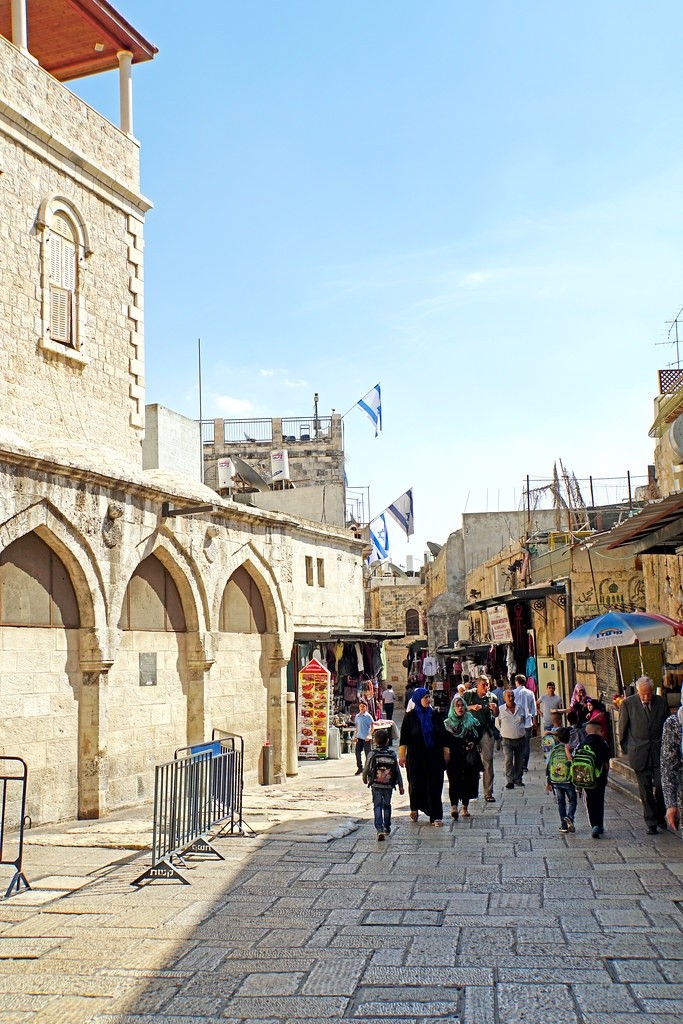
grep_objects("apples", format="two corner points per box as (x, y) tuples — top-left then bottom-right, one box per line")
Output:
(612, 694), (624, 703)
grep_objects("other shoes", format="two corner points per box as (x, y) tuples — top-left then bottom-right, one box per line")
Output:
(646, 821), (658, 834)
(384, 828), (391, 835)
(592, 827), (600, 838)
(600, 828), (604, 834)
(410, 811), (418, 821)
(658, 817), (667, 829)
(506, 783), (514, 788)
(432, 819), (443, 827)
(462, 812), (470, 816)
(377, 831), (385, 841)
(355, 768), (363, 775)
(523, 768), (528, 772)
(564, 816), (575, 833)
(558, 826), (568, 832)
(516, 781), (524, 786)
(451, 811), (458, 819)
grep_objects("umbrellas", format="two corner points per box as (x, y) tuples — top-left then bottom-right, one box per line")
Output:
(557, 609), (683, 699)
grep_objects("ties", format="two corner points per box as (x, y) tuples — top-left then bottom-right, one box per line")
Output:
(644, 705), (651, 723)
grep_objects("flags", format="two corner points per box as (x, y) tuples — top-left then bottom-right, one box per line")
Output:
(385, 489), (414, 537)
(370, 514), (389, 559)
(357, 384), (382, 438)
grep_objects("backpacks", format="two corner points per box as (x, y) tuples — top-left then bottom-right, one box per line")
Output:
(570, 745), (600, 789)
(368, 749), (397, 790)
(542, 734), (556, 759)
(548, 745), (572, 783)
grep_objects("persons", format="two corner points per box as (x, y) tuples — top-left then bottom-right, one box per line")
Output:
(444, 695), (483, 820)
(495, 689), (525, 789)
(454, 675), (499, 802)
(399, 684), (450, 827)
(619, 677), (672, 834)
(353, 702), (374, 775)
(536, 682), (611, 838)
(660, 682), (683, 834)
(363, 730), (405, 841)
(512, 674), (537, 772)
(491, 680), (504, 751)
(382, 685), (396, 720)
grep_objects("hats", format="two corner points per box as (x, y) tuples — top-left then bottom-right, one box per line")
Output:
(587, 699), (598, 708)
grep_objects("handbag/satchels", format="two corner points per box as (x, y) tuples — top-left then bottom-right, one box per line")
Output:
(463, 746), (483, 772)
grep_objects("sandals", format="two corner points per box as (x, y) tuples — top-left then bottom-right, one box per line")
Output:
(485, 795), (496, 802)
